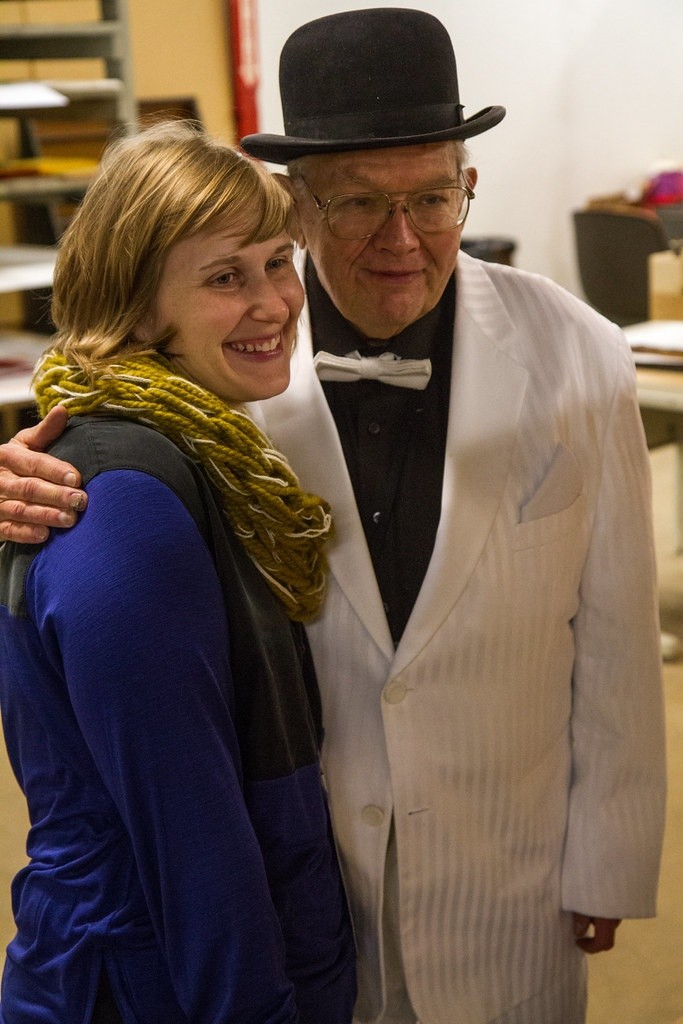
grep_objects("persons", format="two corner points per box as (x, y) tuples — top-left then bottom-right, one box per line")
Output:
(0, 124), (366, 1024)
(0, 4), (666, 1024)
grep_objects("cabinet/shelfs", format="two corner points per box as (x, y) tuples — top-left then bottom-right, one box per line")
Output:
(0, 0), (139, 204)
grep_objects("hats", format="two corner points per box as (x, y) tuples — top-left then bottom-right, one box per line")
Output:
(242, 8), (506, 165)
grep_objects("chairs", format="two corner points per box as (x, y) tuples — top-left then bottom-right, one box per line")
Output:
(573, 212), (669, 329)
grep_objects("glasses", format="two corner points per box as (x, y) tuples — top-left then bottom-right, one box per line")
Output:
(292, 173), (475, 240)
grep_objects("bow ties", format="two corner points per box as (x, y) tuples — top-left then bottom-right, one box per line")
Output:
(314, 350), (432, 389)
